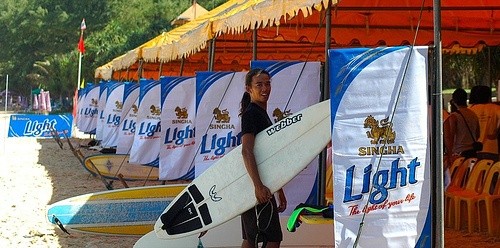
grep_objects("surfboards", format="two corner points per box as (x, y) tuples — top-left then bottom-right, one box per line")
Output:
(47, 185), (189, 237)
(133, 216), (335, 248)
(155, 98), (331, 239)
(83, 154), (159, 180)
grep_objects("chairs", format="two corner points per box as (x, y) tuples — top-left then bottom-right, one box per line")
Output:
(442, 154), (496, 236)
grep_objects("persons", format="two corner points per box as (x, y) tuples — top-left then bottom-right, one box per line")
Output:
(470, 85), (500, 162)
(241, 70), (286, 248)
(443, 100), (452, 150)
(449, 88), (480, 158)
